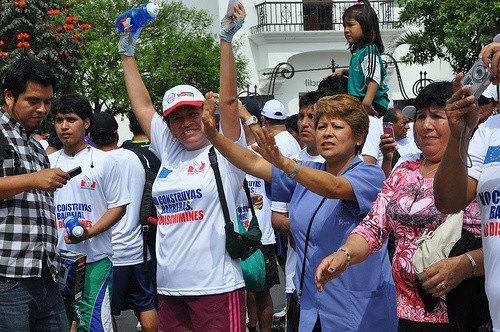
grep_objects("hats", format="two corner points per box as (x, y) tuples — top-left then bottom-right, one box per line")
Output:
(90, 111), (118, 138)
(242, 99), (261, 122)
(260, 99), (286, 120)
(162, 85), (205, 118)
(402, 106), (417, 120)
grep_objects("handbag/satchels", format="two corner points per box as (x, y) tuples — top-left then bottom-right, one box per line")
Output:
(223, 215), (262, 261)
(413, 209), (464, 302)
(236, 218), (266, 291)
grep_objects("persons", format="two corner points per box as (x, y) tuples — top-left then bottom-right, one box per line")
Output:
(117, 2), (248, 332)
(202, 74), (424, 332)
(314, 82), (485, 332)
(34, 106), (162, 332)
(331, 0), (390, 118)
(0, 59), (70, 332)
(46, 93), (132, 332)
(433, 42), (500, 332)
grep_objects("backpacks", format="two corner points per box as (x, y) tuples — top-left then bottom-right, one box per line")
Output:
(122, 141), (157, 259)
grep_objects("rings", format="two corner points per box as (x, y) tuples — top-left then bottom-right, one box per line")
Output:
(440, 282), (447, 290)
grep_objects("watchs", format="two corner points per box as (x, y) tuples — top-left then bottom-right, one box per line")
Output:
(288, 157), (302, 179)
(246, 116), (258, 126)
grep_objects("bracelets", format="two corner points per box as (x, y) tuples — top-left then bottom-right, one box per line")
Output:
(337, 248), (350, 268)
(464, 253), (477, 276)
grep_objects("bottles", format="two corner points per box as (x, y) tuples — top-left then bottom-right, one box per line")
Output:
(62, 215), (85, 238)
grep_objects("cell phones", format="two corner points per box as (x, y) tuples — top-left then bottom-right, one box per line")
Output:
(383, 122), (395, 144)
(67, 166), (82, 178)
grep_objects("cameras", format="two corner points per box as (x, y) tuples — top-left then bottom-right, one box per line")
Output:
(461, 57), (492, 99)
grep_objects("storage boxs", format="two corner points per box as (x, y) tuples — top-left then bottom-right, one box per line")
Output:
(59, 249), (86, 302)
